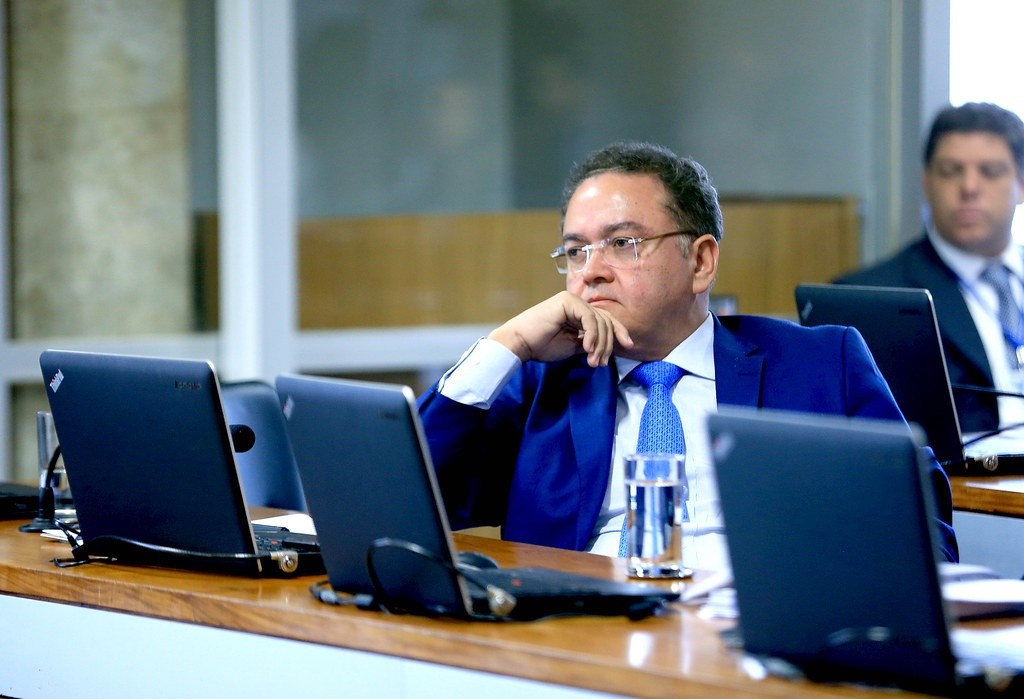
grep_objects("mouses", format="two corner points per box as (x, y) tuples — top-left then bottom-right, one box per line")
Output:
(456, 552), (498, 570)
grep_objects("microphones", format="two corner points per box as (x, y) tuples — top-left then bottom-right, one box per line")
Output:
(230, 424), (256, 454)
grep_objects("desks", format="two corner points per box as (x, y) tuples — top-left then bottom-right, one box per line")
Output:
(1, 477), (1024, 699)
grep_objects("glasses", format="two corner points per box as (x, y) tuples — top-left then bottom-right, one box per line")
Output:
(549, 226), (697, 274)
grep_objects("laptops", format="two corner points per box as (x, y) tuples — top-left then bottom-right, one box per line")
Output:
(792, 284), (1024, 477)
(705, 412), (1024, 699)
(275, 374), (682, 622)
(40, 350), (328, 579)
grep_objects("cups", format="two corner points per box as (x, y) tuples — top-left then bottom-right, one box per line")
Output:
(36, 412), (81, 540)
(624, 453), (689, 579)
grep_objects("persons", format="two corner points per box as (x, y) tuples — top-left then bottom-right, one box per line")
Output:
(830, 102), (1024, 431)
(417, 146), (960, 570)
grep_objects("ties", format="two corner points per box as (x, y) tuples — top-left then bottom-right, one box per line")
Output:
(980, 262), (1023, 371)
(619, 360), (691, 560)
